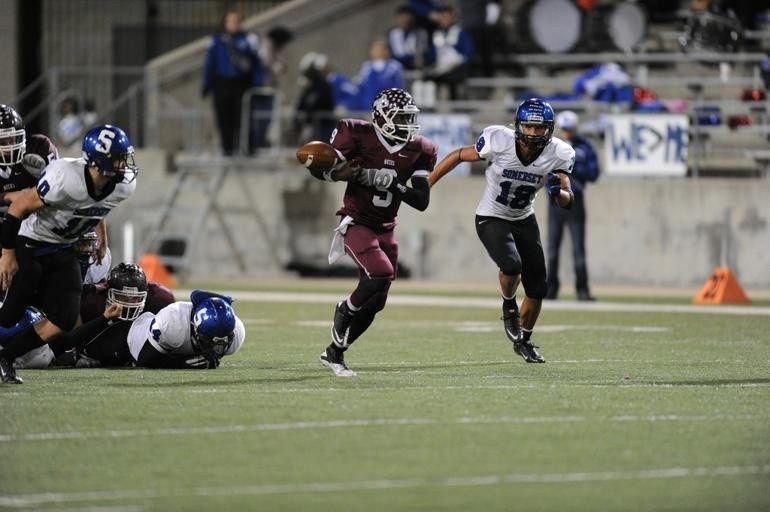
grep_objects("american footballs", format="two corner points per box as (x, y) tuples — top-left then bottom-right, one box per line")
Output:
(296, 141), (346, 169)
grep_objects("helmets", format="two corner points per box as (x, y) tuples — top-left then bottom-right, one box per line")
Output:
(371, 88), (420, 143)
(555, 110), (579, 129)
(0, 104), (26, 166)
(190, 290), (235, 369)
(76, 229), (97, 259)
(82, 125), (138, 183)
(515, 98), (554, 148)
(107, 263), (147, 321)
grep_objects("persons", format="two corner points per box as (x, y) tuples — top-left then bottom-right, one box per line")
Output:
(1, 125), (139, 384)
(199, 2), (769, 156)
(546, 109), (600, 302)
(2, 98), (242, 365)
(429, 97), (576, 365)
(296, 86), (439, 379)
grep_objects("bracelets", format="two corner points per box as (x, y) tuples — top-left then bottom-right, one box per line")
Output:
(321, 167), (337, 182)
(1, 212), (21, 251)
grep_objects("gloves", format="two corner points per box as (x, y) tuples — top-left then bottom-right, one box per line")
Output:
(545, 172), (561, 196)
(358, 168), (396, 187)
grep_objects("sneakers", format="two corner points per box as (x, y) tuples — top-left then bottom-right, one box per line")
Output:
(332, 301), (352, 348)
(0, 346), (23, 385)
(546, 286), (558, 298)
(500, 304), (521, 342)
(514, 341), (545, 363)
(320, 346), (357, 377)
(576, 289), (595, 301)
